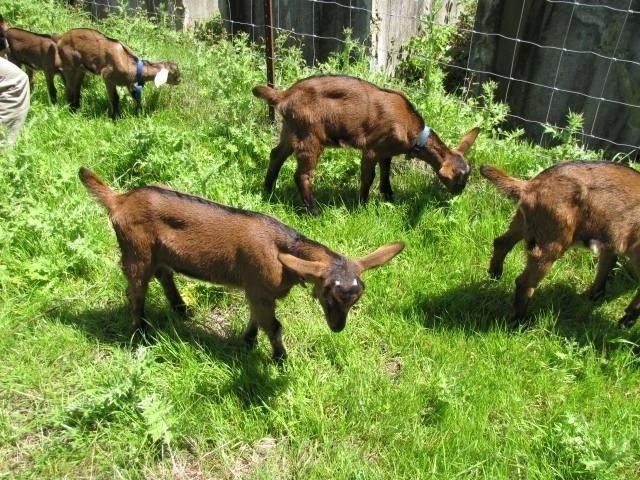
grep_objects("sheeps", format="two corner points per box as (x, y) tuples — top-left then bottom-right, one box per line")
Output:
(252, 73), (481, 216)
(55, 27), (183, 119)
(78, 164), (406, 366)
(479, 157), (640, 327)
(1, 26), (60, 105)
